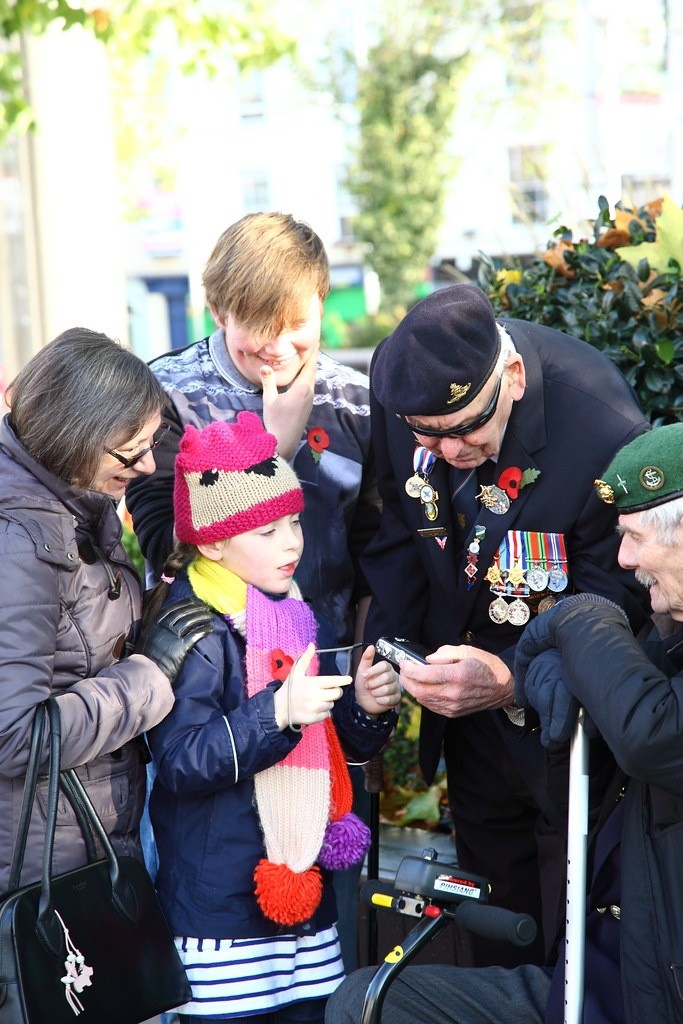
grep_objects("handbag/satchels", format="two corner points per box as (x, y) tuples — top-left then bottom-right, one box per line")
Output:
(3, 701), (193, 1024)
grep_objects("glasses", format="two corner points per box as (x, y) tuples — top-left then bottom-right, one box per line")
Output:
(101, 420), (171, 470)
(393, 370), (508, 439)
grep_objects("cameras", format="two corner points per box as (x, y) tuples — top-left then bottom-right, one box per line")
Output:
(373, 635), (432, 667)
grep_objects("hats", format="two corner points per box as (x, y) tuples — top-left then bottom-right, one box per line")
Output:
(596, 419), (682, 516)
(174, 411), (306, 546)
(370, 284), (499, 418)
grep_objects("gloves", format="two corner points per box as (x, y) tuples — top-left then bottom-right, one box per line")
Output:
(523, 648), (578, 751)
(512, 592), (630, 709)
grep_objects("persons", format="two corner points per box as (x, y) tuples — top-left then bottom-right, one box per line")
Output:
(346, 282), (653, 967)
(327, 418), (683, 1024)
(103, 209), (406, 940)
(2, 326), (218, 1024)
(139, 405), (403, 1021)
